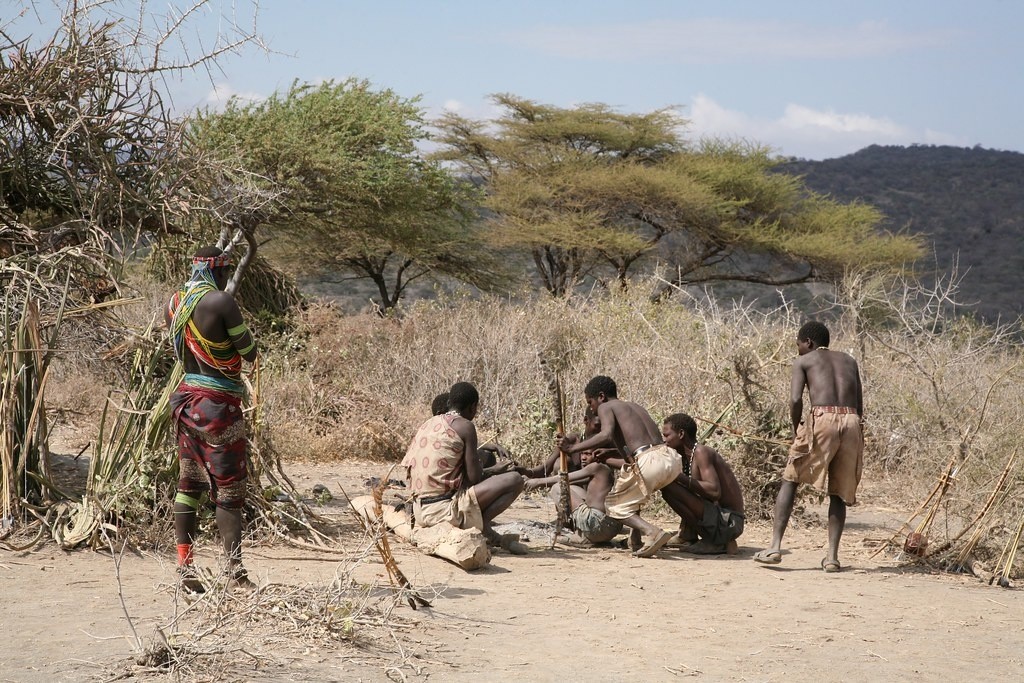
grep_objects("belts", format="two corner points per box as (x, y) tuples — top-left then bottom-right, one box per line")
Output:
(807, 406), (857, 451)
(421, 494), (452, 506)
(632, 443), (662, 457)
(731, 513), (745, 519)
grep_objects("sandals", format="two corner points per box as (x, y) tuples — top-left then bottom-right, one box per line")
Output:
(553, 533), (594, 548)
(754, 549), (782, 563)
(821, 557), (840, 572)
(627, 527), (642, 551)
(631, 529), (672, 558)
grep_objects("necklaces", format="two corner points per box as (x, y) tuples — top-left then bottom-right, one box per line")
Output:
(683, 442), (698, 477)
(580, 432), (585, 442)
(815, 346), (830, 350)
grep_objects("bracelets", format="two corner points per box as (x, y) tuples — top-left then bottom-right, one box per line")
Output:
(688, 477), (691, 490)
(529, 468), (535, 478)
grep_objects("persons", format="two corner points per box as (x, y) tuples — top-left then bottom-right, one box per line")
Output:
(430, 391), (512, 525)
(507, 401), (614, 479)
(524, 447), (622, 548)
(405, 382), (525, 545)
(557, 376), (682, 558)
(659, 413), (746, 555)
(752, 323), (864, 573)
(164, 246), (258, 590)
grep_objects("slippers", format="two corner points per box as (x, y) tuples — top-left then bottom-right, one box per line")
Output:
(679, 541), (727, 554)
(663, 539), (691, 547)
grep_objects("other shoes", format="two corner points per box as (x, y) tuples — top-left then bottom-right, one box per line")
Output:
(480, 529), (502, 547)
